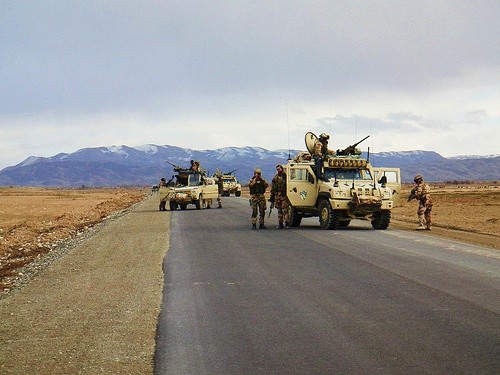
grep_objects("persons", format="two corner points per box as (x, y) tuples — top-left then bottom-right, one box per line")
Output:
(312, 132), (335, 158)
(268, 164), (292, 230)
(195, 160), (200, 172)
(248, 169), (269, 230)
(189, 160), (196, 171)
(158, 178), (168, 211)
(215, 176), (223, 209)
(404, 173), (434, 230)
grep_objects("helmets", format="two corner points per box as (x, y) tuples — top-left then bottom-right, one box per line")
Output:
(320, 133), (330, 140)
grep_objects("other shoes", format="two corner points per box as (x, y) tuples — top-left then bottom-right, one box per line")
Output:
(416, 225), (432, 230)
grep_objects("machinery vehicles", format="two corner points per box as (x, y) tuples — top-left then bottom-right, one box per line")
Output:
(165, 161), (218, 210)
(212, 167), (242, 197)
(276, 133), (402, 231)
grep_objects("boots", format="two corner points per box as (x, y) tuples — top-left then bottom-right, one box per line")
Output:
(252, 221), (290, 230)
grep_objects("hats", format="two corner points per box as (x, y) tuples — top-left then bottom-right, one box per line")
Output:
(413, 175), (423, 181)
(254, 169), (261, 174)
